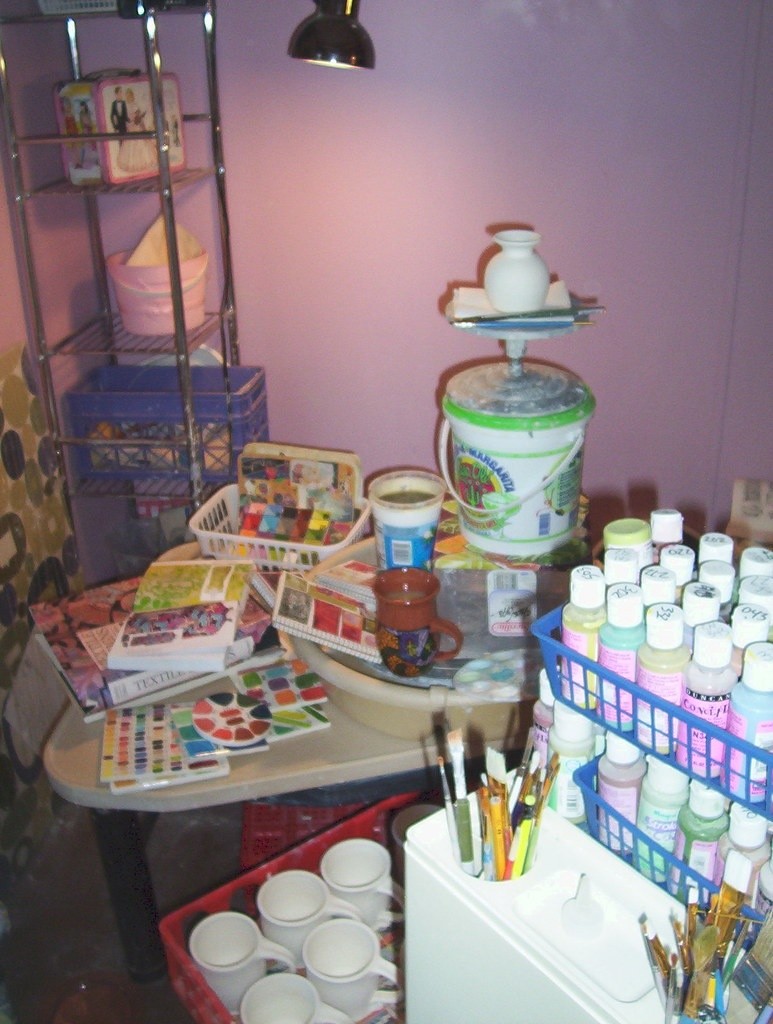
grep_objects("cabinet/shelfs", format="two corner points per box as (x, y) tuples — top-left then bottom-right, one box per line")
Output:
(0, 0), (239, 540)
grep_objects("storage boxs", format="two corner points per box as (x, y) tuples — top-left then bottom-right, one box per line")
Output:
(67, 365), (270, 481)
(157, 790), (461, 1024)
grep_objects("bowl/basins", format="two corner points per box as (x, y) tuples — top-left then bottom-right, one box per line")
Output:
(293, 635), (540, 742)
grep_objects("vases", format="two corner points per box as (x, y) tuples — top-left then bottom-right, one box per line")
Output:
(485, 230), (550, 313)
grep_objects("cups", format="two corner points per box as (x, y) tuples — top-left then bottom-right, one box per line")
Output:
(368, 470), (447, 574)
(256, 870), (361, 968)
(320, 838), (405, 931)
(392, 804), (445, 875)
(372, 569), (463, 677)
(189, 911), (297, 1015)
(240, 972), (354, 1024)
(302, 919), (403, 1018)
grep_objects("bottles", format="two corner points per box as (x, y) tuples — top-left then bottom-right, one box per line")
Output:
(483, 230), (549, 314)
(533, 509), (773, 942)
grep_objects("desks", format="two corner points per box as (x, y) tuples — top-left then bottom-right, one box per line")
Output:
(406, 762), (745, 1024)
(44, 638), (530, 981)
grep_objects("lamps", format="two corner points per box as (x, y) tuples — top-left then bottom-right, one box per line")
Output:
(287, 0), (377, 69)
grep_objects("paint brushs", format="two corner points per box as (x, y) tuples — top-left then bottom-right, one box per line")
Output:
(437, 728), (561, 880)
(639, 848), (764, 1024)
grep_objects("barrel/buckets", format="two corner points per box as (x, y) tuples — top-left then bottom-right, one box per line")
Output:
(107, 247), (209, 335)
(437, 382), (597, 556)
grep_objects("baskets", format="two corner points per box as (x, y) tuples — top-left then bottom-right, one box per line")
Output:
(572, 753), (765, 950)
(531, 605), (773, 822)
(188, 483), (372, 576)
